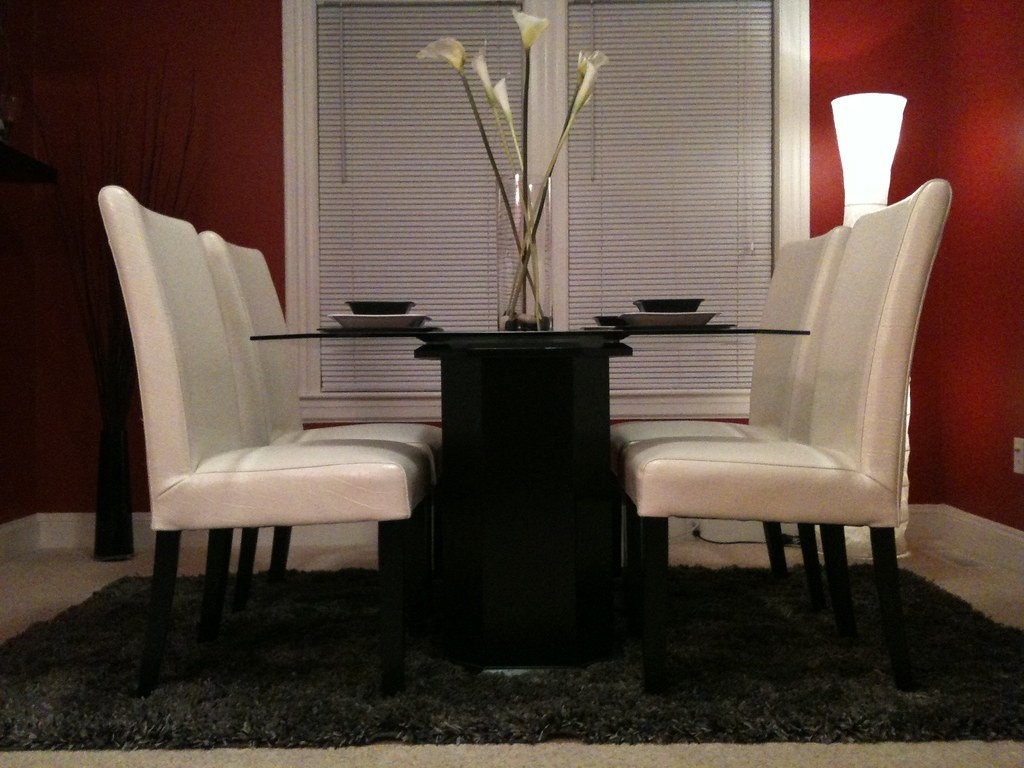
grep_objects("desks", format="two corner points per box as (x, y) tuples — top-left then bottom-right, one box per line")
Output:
(250, 322), (811, 673)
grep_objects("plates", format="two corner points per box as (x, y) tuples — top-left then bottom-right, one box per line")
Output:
(579, 326), (615, 331)
(316, 326), (444, 333)
(613, 323), (738, 330)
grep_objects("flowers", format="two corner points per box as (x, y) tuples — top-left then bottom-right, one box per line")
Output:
(415, 9), (609, 176)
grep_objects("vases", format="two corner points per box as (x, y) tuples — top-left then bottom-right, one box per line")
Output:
(496, 174), (553, 328)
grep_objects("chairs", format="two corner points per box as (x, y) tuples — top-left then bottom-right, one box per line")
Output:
(619, 176), (952, 694)
(100, 186), (437, 693)
(199, 230), (443, 612)
(610, 225), (851, 614)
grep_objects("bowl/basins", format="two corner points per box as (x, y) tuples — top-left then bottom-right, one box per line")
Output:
(345, 300), (416, 314)
(633, 298), (705, 312)
(328, 314), (433, 327)
(592, 315), (618, 326)
(618, 313), (721, 324)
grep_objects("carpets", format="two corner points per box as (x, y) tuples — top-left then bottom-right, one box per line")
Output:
(1, 562), (1024, 749)
